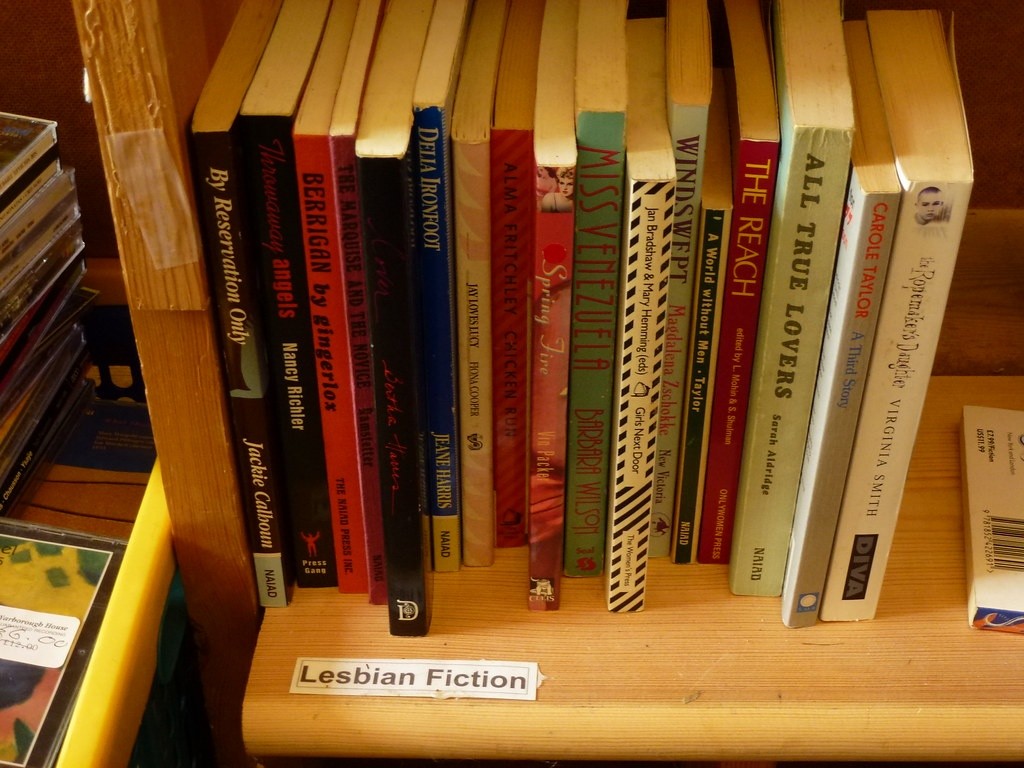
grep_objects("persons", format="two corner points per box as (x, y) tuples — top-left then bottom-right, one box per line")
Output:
(912, 184), (944, 225)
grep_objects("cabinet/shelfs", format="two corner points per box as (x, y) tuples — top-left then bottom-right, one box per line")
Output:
(72, 0), (1023, 767)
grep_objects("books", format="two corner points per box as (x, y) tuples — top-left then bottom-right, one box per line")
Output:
(188, 1), (1024, 640)
(0, 103), (107, 534)
